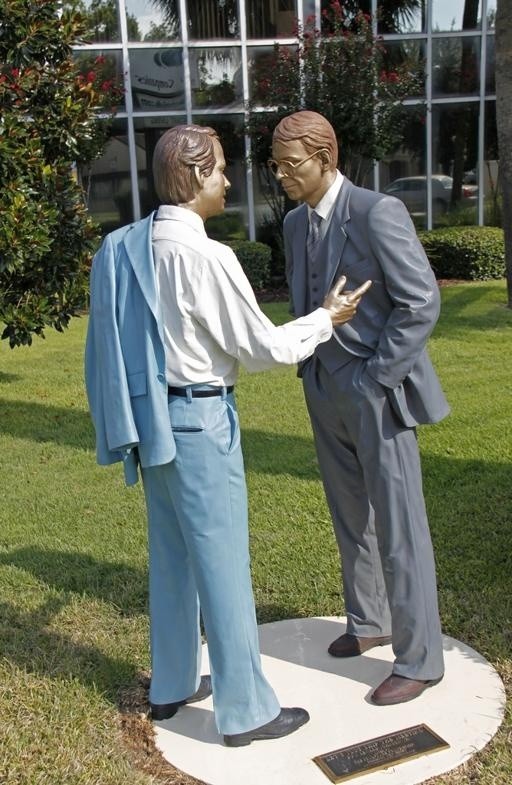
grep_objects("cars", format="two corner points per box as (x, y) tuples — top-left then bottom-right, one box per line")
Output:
(382, 175), (478, 218)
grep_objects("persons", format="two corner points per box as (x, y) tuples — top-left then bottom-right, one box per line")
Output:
(84, 123), (373, 746)
(266, 111), (452, 706)
